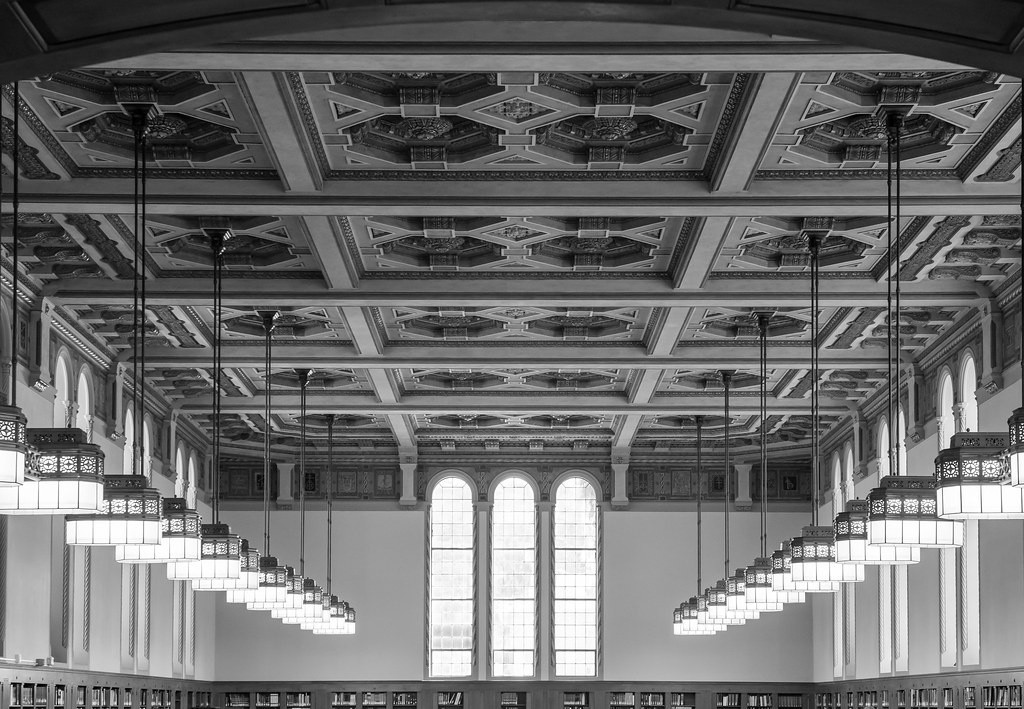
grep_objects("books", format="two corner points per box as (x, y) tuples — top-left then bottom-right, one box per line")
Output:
(0, 686), (1024, 709)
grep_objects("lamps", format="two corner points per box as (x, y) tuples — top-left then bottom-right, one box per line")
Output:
(0, 72), (356, 636)
(674, 80), (1023, 637)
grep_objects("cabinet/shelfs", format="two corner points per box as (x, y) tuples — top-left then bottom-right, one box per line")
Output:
(0, 661), (1024, 709)
